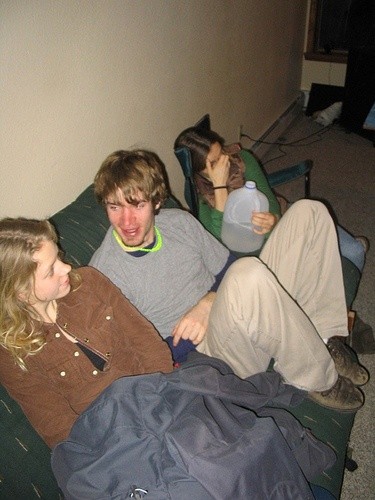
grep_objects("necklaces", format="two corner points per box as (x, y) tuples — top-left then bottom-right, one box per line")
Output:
(112, 225), (163, 254)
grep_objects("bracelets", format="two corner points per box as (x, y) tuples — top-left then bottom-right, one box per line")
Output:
(211, 185), (229, 191)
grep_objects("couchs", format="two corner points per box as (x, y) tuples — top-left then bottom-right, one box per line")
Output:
(0, 182), (368, 500)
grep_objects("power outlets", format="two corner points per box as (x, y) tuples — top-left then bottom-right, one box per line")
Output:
(238, 125), (243, 140)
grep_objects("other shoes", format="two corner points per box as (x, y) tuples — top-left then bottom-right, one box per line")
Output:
(325, 337), (369, 386)
(306, 376), (365, 415)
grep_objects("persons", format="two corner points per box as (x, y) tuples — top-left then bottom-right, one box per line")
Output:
(173, 121), (369, 276)
(83, 147), (371, 414)
(0, 215), (178, 452)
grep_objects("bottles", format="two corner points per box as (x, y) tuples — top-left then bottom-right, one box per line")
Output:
(221, 181), (270, 253)
